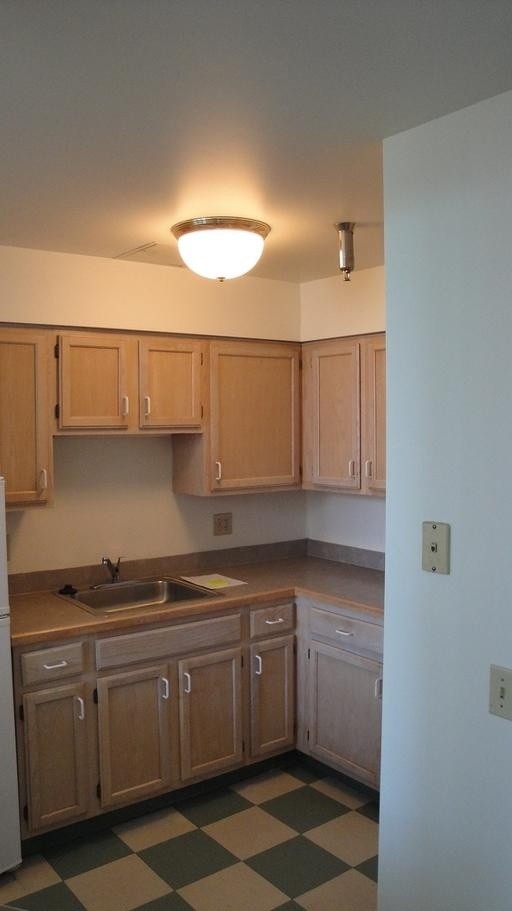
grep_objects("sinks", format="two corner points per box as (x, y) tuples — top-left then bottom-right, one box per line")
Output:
(52, 576), (227, 616)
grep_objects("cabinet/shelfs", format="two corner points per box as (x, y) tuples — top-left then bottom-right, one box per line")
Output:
(300, 332), (386, 499)
(11, 633), (97, 840)
(294, 593), (384, 793)
(0, 322), (55, 504)
(55, 325), (205, 436)
(172, 332), (302, 498)
(93, 605), (247, 815)
(246, 596), (297, 766)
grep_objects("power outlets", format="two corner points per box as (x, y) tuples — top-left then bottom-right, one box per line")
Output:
(169, 215), (272, 283)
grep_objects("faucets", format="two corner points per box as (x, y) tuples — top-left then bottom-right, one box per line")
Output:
(100, 555), (121, 582)
